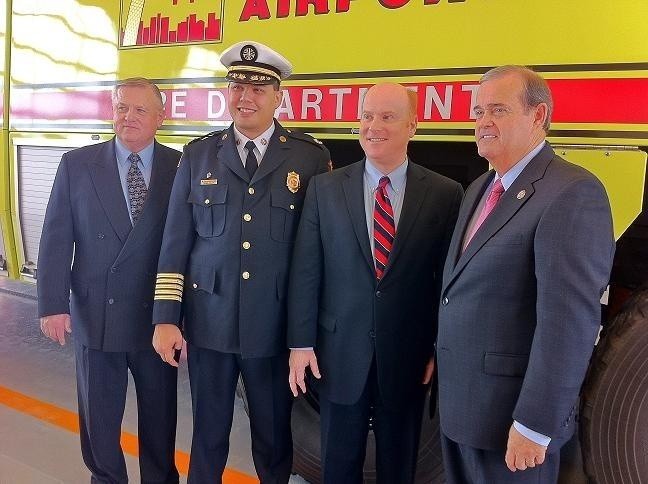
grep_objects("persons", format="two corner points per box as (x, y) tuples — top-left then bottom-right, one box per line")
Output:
(35, 77), (180, 484)
(430, 65), (617, 484)
(152, 40), (332, 484)
(287, 81), (465, 484)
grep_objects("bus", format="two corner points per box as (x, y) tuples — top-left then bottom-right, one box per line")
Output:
(0, 0), (648, 484)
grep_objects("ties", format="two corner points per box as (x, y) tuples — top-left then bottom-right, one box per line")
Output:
(462, 179), (504, 254)
(126, 154), (147, 225)
(373, 177), (396, 279)
(245, 141), (258, 179)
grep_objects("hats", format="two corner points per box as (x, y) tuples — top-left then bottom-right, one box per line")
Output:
(219, 40), (293, 85)
